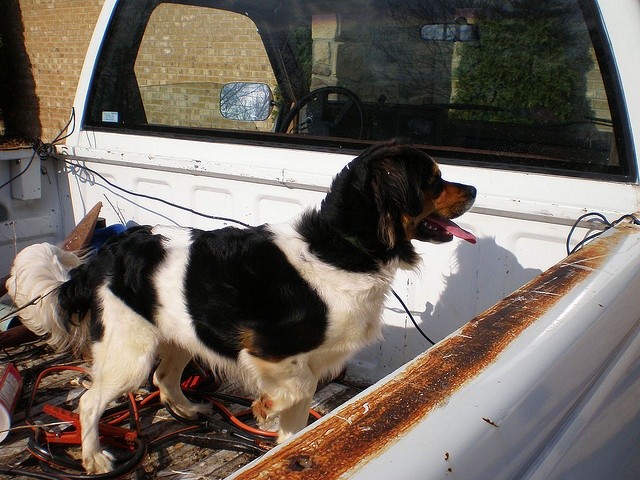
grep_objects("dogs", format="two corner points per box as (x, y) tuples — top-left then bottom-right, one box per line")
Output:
(4, 137), (477, 476)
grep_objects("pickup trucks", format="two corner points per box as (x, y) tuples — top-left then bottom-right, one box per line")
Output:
(0, 1), (639, 480)
